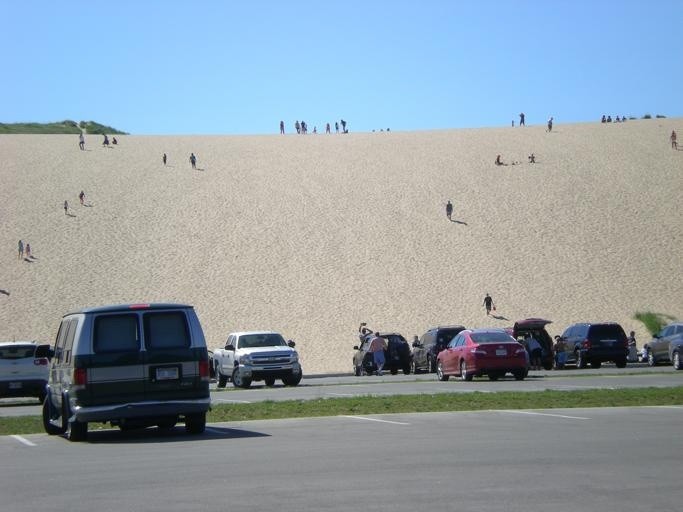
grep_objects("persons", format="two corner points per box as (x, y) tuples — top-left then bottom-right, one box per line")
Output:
(519, 112), (525, 125)
(358, 323), (373, 344)
(545, 117), (554, 133)
(102, 133), (109, 147)
(671, 129), (677, 147)
(446, 200), (453, 221)
(369, 332), (387, 376)
(162, 153), (167, 165)
(601, 115), (627, 125)
(79, 190), (85, 204)
(112, 136), (117, 144)
(78, 133), (85, 150)
(627, 331), (639, 362)
(523, 334), (543, 370)
(189, 152), (197, 169)
(279, 118), (347, 135)
(482, 293), (494, 315)
(26, 244), (30, 256)
(412, 335), (420, 348)
(640, 342), (648, 362)
(495, 155), (503, 166)
(18, 239), (24, 256)
(63, 200), (68, 214)
(553, 338), (568, 369)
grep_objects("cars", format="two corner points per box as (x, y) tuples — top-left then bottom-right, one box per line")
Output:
(641, 319), (683, 371)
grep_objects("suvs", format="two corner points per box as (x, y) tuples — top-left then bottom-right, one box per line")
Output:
(349, 318), (632, 381)
(0, 339), (52, 405)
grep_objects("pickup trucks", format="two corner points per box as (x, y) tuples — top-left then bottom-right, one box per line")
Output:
(210, 330), (304, 390)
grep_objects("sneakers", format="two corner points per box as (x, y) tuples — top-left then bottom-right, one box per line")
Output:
(376, 370), (383, 376)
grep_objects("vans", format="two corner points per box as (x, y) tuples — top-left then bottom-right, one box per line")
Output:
(38, 299), (219, 447)
